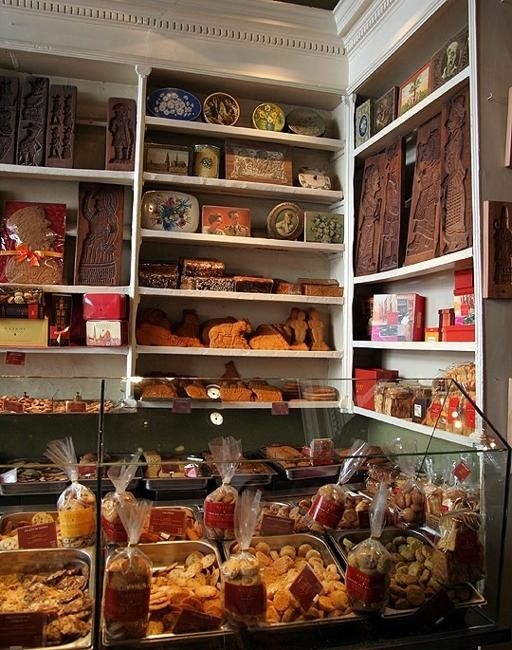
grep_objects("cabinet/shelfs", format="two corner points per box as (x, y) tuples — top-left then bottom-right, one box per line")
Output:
(0, 0), (511, 650)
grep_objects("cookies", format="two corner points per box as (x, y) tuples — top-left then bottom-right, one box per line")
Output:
(0, 490), (95, 646)
(101, 466), (480, 642)
